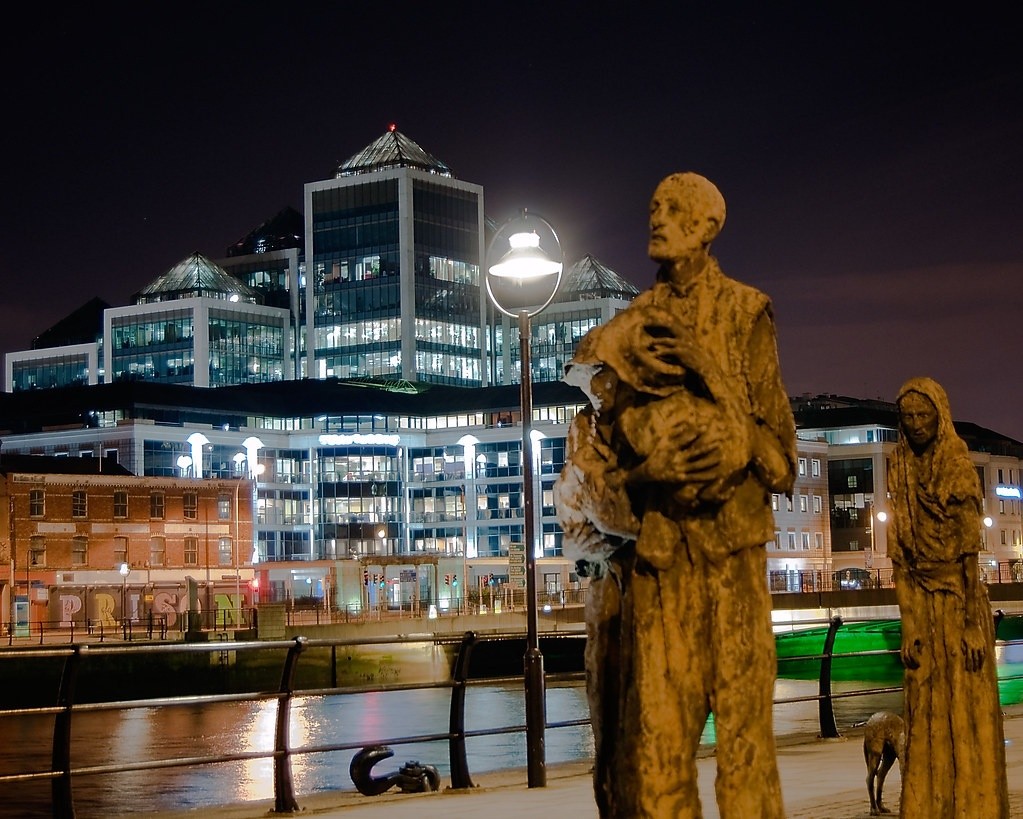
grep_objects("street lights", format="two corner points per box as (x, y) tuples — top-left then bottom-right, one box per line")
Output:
(120, 562), (129, 618)
(233, 464), (267, 629)
(481, 203), (567, 788)
(377, 529), (388, 611)
(870, 501), (888, 568)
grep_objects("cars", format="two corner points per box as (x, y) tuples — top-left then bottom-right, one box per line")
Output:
(830, 566), (882, 589)
(979, 567), (988, 582)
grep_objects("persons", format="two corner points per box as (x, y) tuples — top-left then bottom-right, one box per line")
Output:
(887, 375), (1010, 819)
(596, 165), (802, 819)
(555, 321), (710, 816)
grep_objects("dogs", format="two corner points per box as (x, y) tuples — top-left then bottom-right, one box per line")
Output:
(863, 710), (906, 816)
